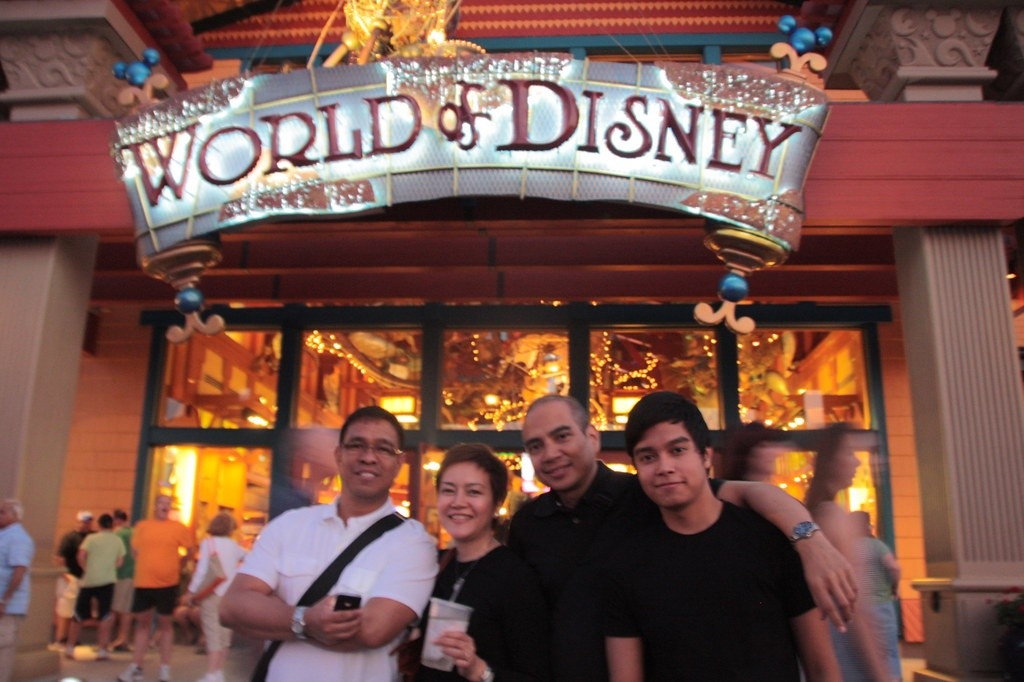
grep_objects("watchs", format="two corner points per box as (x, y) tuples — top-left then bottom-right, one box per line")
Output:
(481, 667), (495, 682)
(789, 522), (820, 541)
(290, 605), (308, 641)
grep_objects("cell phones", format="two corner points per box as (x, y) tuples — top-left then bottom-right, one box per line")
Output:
(334, 594), (362, 612)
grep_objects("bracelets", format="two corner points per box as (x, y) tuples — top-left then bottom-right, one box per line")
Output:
(0, 600), (7, 604)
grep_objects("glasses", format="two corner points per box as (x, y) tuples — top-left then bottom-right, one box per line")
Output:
(341, 440), (403, 459)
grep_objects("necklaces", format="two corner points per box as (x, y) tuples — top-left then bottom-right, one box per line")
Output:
(448, 531), (491, 601)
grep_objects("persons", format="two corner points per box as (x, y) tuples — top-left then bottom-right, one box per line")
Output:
(405, 440), (553, 682)
(600, 391), (845, 682)
(715, 419), (905, 682)
(0, 496), (35, 682)
(219, 405), (442, 682)
(50, 495), (205, 682)
(177, 512), (248, 682)
(503, 394), (858, 682)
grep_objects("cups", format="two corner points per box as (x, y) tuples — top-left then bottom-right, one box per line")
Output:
(421, 596), (474, 673)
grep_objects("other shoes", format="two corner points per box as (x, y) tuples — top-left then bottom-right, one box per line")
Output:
(96, 649), (108, 660)
(118, 664), (143, 682)
(159, 664), (170, 681)
(198, 672), (223, 682)
